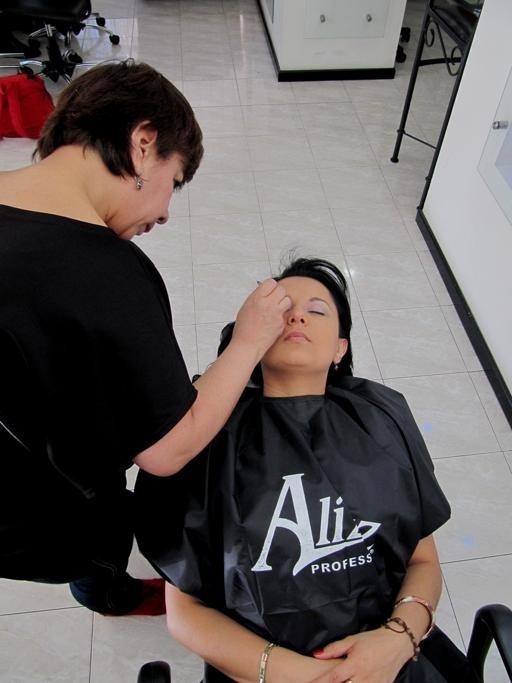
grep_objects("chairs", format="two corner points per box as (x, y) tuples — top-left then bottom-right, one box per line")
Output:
(0, 0), (124, 83)
(132, 319), (511, 681)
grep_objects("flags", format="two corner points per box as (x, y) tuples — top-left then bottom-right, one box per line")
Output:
(383, 613), (421, 664)
(393, 592), (437, 643)
(259, 636), (280, 683)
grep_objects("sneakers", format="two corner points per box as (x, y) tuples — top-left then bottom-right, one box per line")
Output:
(101, 579), (166, 616)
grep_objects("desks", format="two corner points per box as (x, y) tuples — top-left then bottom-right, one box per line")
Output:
(388, 0), (486, 219)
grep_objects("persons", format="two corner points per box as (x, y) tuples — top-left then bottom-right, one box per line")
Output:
(0, 55), (294, 617)
(130, 254), (481, 683)
